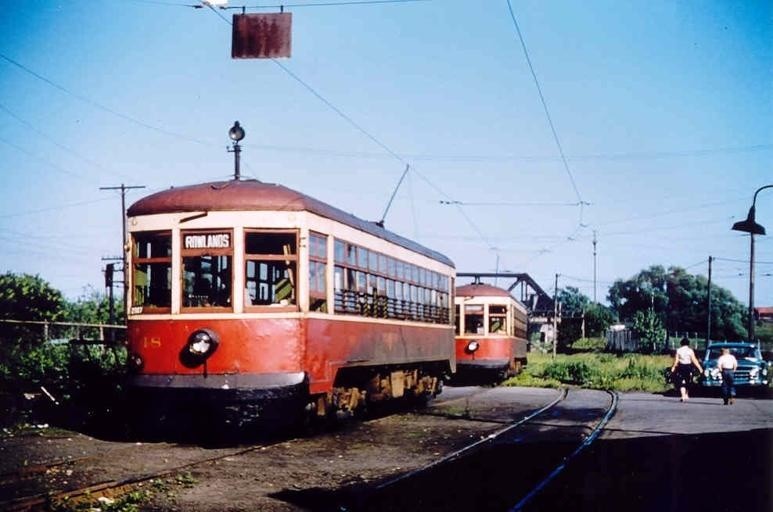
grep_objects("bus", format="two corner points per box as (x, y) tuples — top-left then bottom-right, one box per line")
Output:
(122, 118), (456, 432)
(442, 278), (529, 387)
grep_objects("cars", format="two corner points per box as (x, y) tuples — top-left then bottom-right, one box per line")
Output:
(697, 339), (768, 397)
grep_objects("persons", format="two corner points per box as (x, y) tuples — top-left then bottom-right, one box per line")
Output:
(217, 268), (253, 308)
(718, 346), (739, 406)
(666, 337), (706, 403)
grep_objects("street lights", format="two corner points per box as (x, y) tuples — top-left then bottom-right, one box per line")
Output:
(729, 185), (772, 235)
